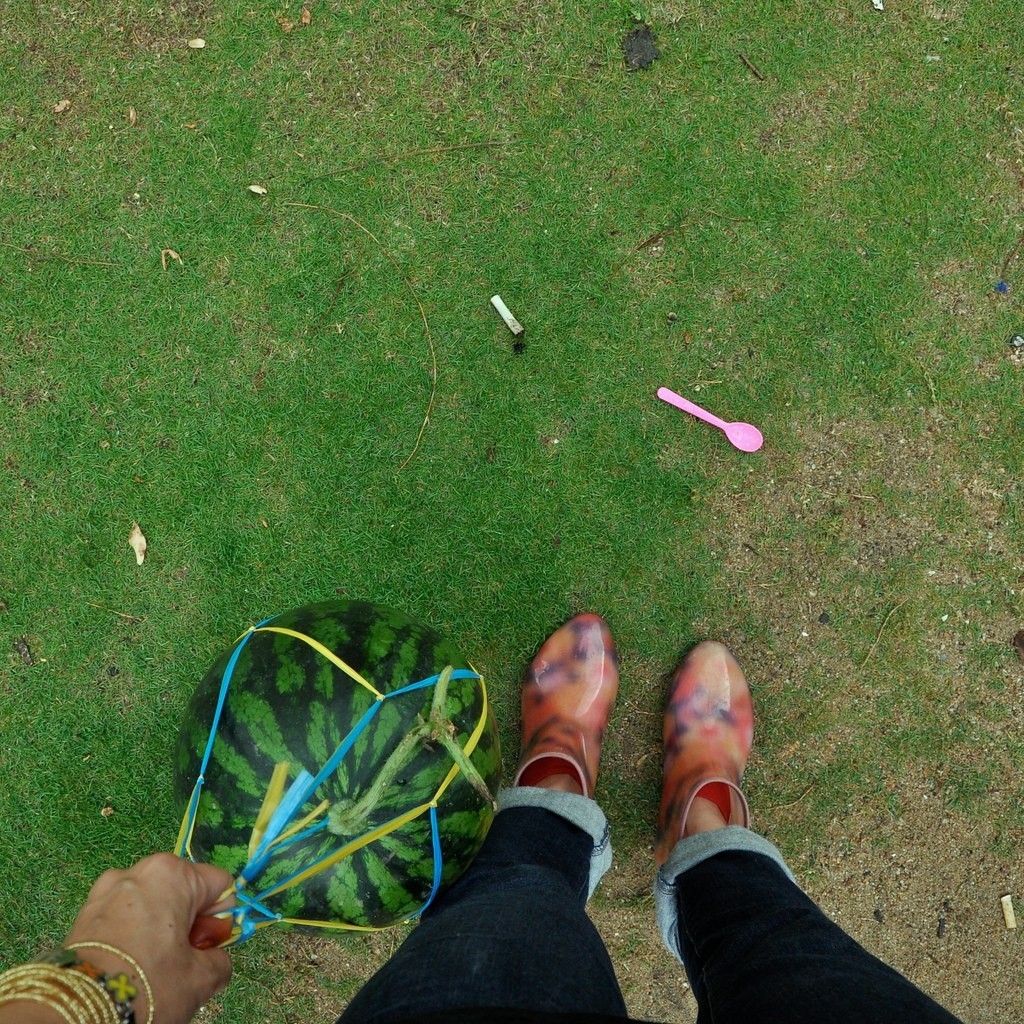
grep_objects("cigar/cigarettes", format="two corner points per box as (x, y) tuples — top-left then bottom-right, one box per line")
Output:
(490, 295), (526, 334)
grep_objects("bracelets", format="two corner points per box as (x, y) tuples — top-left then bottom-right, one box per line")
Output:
(0, 941), (156, 1024)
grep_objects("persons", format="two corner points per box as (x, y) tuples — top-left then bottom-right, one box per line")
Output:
(0, 614), (975, 1024)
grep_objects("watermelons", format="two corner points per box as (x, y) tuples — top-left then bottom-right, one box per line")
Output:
(170, 600), (503, 938)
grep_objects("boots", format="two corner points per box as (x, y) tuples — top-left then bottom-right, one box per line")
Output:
(510, 612), (620, 798)
(654, 641), (756, 868)
